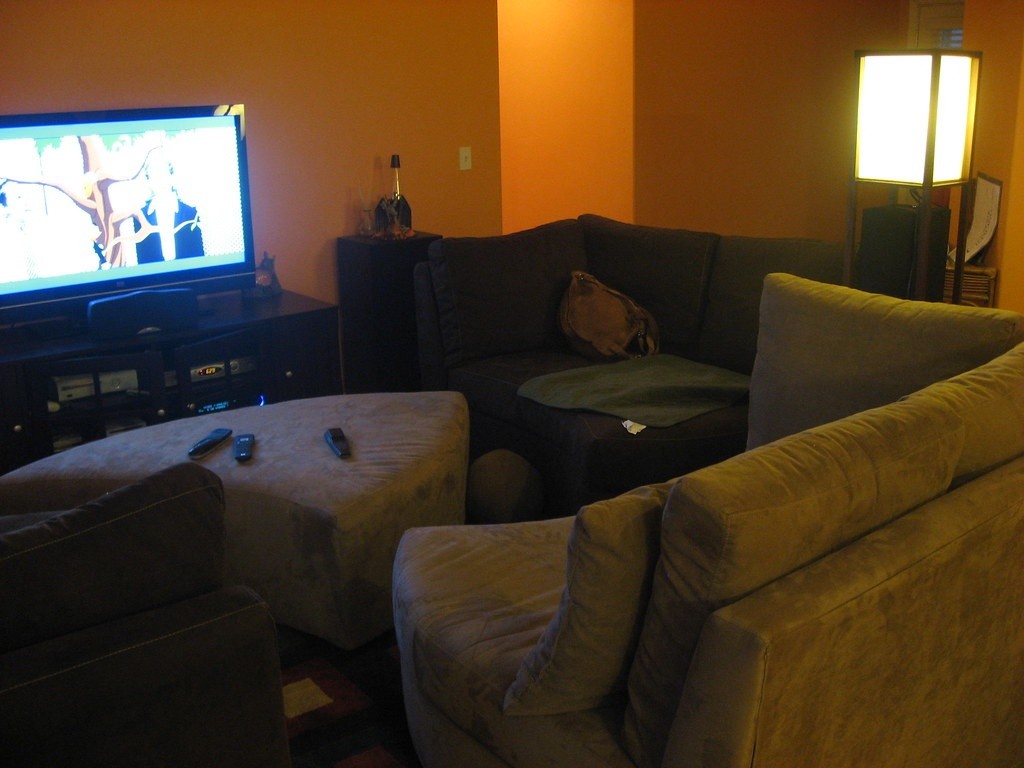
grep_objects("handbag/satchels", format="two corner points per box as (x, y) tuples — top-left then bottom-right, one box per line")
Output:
(559, 271), (659, 362)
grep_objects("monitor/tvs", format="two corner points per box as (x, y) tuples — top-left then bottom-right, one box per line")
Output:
(0, 103), (256, 325)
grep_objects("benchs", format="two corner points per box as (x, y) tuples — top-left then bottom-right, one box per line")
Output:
(0, 389), (468, 653)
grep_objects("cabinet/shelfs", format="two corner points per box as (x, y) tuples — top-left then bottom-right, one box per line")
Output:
(0, 287), (339, 475)
(338, 232), (443, 392)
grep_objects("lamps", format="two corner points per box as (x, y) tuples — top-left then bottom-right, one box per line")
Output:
(844, 48), (984, 302)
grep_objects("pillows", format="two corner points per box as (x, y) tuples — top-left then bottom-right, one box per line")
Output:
(501, 476), (678, 718)
(467, 450), (535, 522)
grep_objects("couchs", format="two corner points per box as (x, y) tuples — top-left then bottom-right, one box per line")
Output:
(414, 215), (846, 518)
(0, 461), (290, 768)
(390, 274), (1024, 768)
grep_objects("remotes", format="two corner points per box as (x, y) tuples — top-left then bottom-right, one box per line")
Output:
(233, 434), (254, 462)
(188, 428), (232, 459)
(324, 428), (352, 458)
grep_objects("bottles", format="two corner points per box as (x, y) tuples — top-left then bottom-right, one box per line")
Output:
(357, 208), (376, 237)
